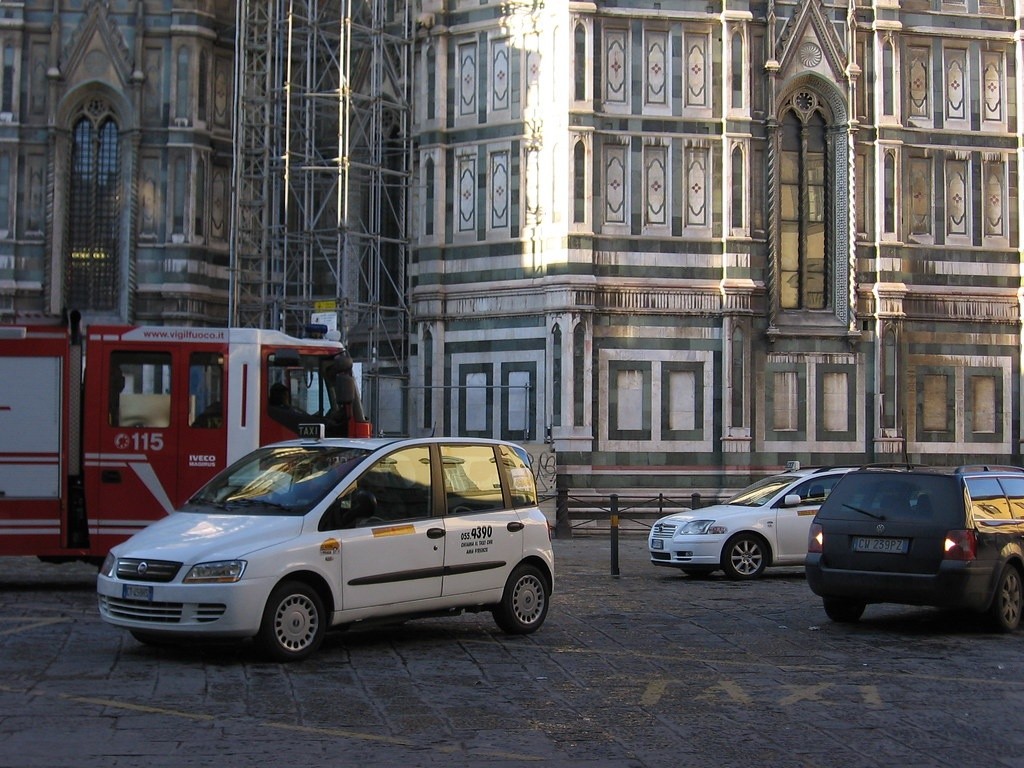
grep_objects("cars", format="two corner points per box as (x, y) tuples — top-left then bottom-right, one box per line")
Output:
(647, 461), (903, 580)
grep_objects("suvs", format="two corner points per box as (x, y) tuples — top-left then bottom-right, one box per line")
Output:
(804, 461), (1024, 631)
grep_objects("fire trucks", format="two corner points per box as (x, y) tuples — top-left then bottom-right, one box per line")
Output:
(1, 310), (374, 563)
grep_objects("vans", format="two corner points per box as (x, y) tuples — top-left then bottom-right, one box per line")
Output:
(95, 435), (557, 662)
